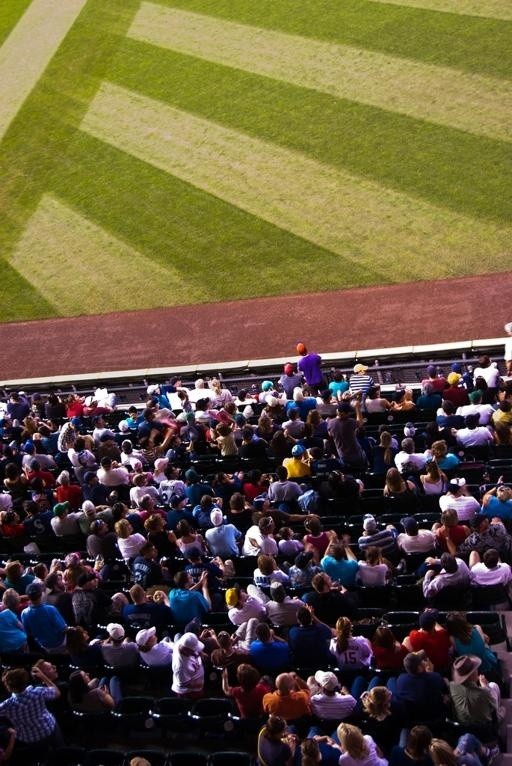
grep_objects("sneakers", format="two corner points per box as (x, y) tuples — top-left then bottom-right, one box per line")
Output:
(485, 747), (499, 765)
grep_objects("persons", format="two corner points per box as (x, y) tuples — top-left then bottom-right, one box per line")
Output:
(2, 321), (511, 765)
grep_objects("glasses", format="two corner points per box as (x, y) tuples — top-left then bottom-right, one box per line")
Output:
(217, 634), (227, 639)
(260, 515), (272, 529)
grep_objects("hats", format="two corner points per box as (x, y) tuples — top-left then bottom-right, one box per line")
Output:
(253, 491), (268, 509)
(65, 553), (80, 567)
(182, 617), (206, 652)
(290, 444), (304, 455)
(106, 623), (124, 640)
(353, 364), (369, 372)
(270, 578), (286, 601)
(72, 418), (83, 430)
(226, 588), (239, 607)
(339, 403), (354, 412)
(403, 649), (424, 671)
(294, 551), (312, 569)
(261, 343), (306, 421)
(31, 393), (40, 400)
(25, 441), (34, 454)
(135, 627), (156, 647)
(361, 513), (375, 531)
(470, 513), (486, 528)
(366, 386), (377, 395)
(391, 389), (403, 400)
(447, 478), (466, 493)
(314, 670), (340, 692)
(183, 546), (200, 560)
(419, 609), (438, 627)
(427, 363), (484, 428)
(275, 672), (296, 690)
(400, 517), (416, 528)
(0, 419), (4, 426)
(452, 654), (481, 686)
(52, 469), (98, 517)
(77, 574), (96, 587)
(118, 375), (250, 431)
(27, 583), (44, 597)
(121, 433), (198, 508)
(210, 508), (223, 526)
